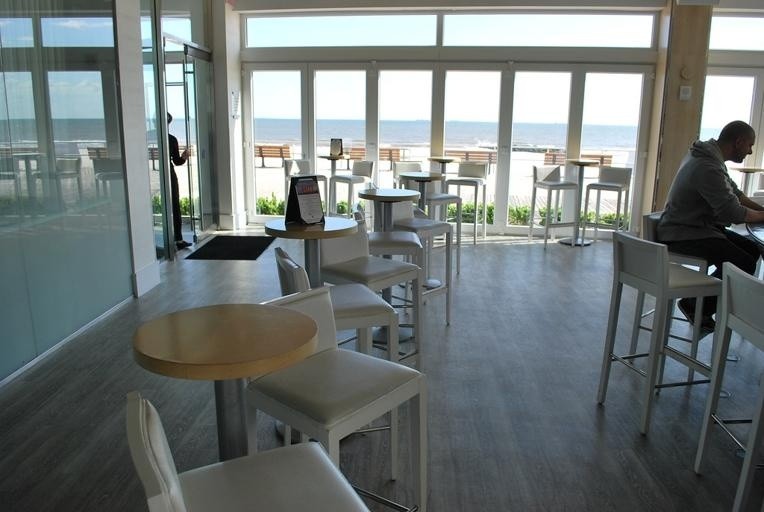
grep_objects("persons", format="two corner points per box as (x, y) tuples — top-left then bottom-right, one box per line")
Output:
(655, 120), (763, 333)
(166, 112), (192, 249)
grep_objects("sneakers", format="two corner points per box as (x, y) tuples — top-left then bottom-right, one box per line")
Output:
(176, 241), (192, 247)
(677, 298), (715, 332)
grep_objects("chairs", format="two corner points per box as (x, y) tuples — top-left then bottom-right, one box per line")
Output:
(1, 155), (124, 226)
